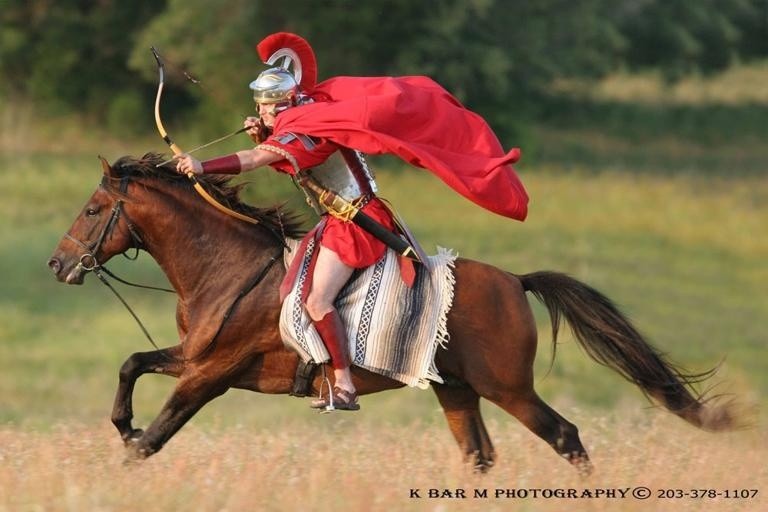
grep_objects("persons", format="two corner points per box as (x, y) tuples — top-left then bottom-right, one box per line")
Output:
(172, 31), (400, 411)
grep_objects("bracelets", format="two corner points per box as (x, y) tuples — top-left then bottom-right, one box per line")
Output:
(200, 154), (241, 174)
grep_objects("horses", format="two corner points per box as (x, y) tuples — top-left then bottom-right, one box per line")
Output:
(44, 151), (759, 481)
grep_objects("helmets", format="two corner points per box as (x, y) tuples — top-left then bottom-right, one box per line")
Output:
(249, 66), (298, 117)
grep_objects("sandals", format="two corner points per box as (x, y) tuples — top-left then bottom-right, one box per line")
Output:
(310, 386), (360, 411)
